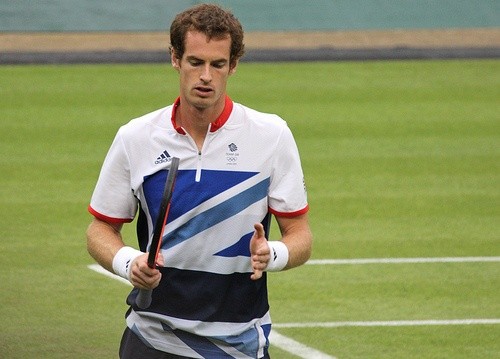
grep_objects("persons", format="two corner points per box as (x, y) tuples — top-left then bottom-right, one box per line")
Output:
(85, 4), (311, 359)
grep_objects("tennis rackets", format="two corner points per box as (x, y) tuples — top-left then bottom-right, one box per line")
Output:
(136, 156), (179, 308)
